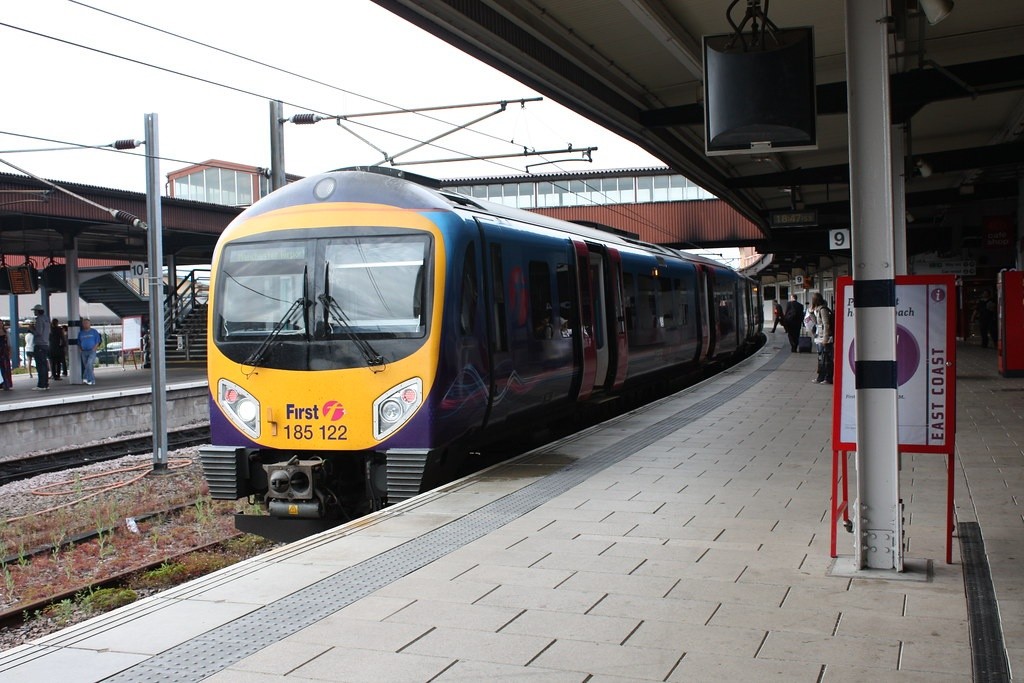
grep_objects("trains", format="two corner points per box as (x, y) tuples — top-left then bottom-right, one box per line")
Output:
(198, 165), (765, 542)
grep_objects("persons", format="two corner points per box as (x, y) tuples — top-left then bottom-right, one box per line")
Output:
(48, 318), (68, 380)
(24, 327), (37, 378)
(804, 292), (834, 384)
(78, 319), (100, 385)
(784, 295), (803, 352)
(0, 319), (14, 390)
(970, 288), (998, 347)
(29, 304), (50, 391)
(770, 300), (787, 333)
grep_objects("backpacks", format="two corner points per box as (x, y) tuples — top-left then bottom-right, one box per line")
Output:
(819, 307), (834, 335)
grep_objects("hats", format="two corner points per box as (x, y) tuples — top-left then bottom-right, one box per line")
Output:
(31, 305), (43, 310)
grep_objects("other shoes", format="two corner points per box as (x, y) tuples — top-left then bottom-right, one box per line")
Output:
(769, 331), (774, 333)
(29, 375), (32, 378)
(820, 380), (831, 384)
(93, 380), (95, 384)
(87, 381), (92, 385)
(83, 379), (87, 384)
(812, 379), (819, 383)
(55, 378), (62, 381)
(47, 387), (50, 389)
(32, 386), (46, 390)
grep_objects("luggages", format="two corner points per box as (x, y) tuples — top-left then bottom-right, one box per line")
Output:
(798, 326), (812, 354)
